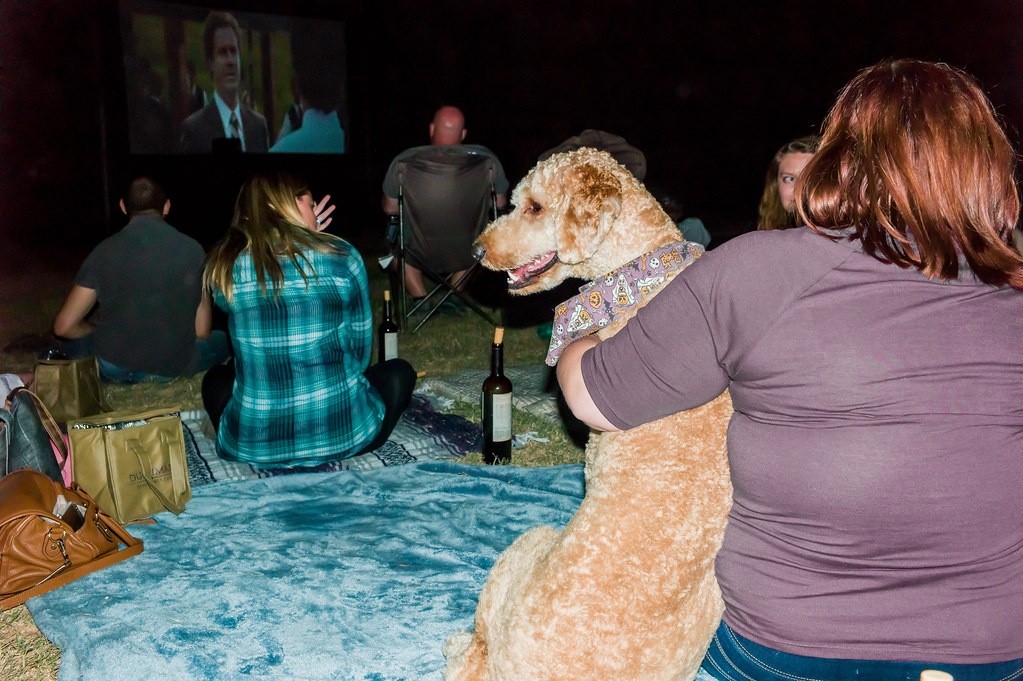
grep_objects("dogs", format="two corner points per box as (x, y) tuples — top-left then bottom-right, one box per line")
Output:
(439, 142), (734, 681)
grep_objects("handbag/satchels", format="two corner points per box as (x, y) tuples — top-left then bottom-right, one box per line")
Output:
(34, 355), (103, 423)
(67, 405), (192, 525)
(0, 386), (68, 488)
(0, 469), (143, 613)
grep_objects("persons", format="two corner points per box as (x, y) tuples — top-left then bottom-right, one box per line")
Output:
(755, 133), (826, 234)
(382, 108), (509, 314)
(0, 372), (34, 408)
(557, 62), (1023, 681)
(644, 187), (712, 250)
(48, 174), (235, 386)
(201, 174), (416, 472)
(164, 10), (348, 157)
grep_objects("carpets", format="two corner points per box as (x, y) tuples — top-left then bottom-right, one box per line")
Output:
(422, 368), (570, 418)
(182, 383), (548, 486)
(27, 460), (582, 680)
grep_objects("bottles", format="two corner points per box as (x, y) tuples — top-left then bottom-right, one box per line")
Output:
(377, 290), (399, 363)
(482, 326), (513, 465)
(919, 669), (953, 681)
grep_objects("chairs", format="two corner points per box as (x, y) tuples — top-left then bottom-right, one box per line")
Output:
(390, 149), (506, 332)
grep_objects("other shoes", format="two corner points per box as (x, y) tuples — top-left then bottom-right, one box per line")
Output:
(403, 304), (438, 319)
(440, 300), (466, 315)
